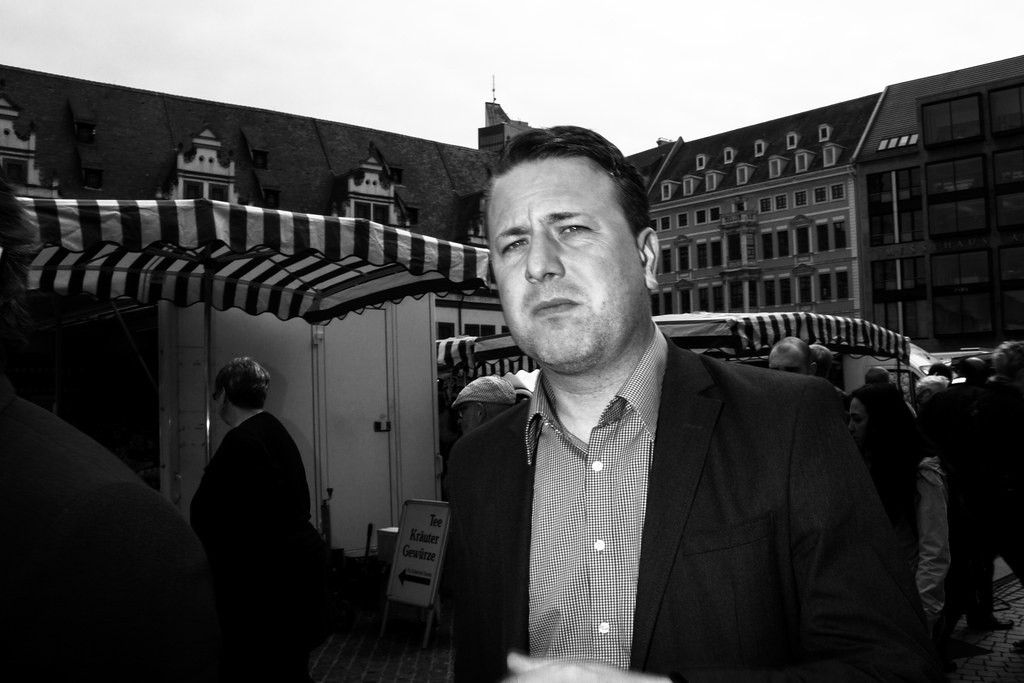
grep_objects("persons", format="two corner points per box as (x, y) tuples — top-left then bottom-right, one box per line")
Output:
(0, 356), (335, 683)
(454, 336), (1024, 670)
(444, 126), (936, 683)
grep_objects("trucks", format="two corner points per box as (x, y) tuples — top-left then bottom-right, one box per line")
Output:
(732, 338), (995, 403)
(155, 291), (438, 565)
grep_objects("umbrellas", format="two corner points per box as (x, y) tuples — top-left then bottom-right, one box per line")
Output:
(7, 197), (1024, 464)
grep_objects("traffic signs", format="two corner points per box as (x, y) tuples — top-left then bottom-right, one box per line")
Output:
(386, 498), (451, 606)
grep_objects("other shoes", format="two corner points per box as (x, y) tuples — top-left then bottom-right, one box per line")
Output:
(995, 619), (1015, 629)
(1012, 638), (1024, 648)
(938, 657), (958, 673)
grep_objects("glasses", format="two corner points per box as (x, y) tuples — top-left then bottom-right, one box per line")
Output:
(456, 403), (475, 418)
(212, 388), (221, 401)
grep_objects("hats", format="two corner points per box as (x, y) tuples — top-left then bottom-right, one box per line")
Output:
(450, 375), (516, 409)
